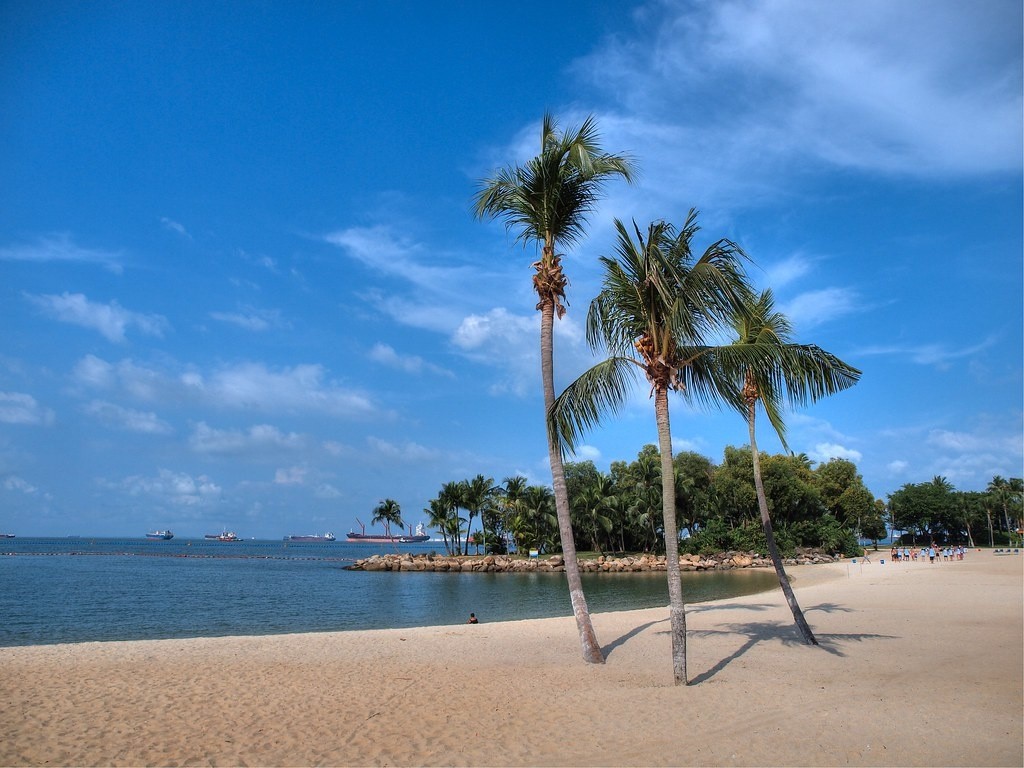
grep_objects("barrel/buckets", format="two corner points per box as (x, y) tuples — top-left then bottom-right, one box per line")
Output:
(881, 560), (884, 564)
(852, 560), (856, 563)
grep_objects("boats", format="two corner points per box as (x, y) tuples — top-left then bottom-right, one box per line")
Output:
(345, 516), (430, 543)
(145, 529), (174, 542)
(283, 533), (336, 541)
(204, 529), (244, 541)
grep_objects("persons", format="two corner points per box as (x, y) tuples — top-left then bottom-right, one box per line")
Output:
(467, 613), (478, 624)
(861, 544), (965, 564)
